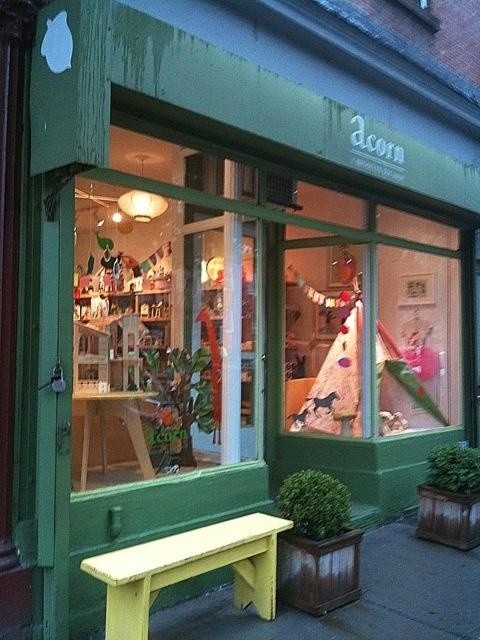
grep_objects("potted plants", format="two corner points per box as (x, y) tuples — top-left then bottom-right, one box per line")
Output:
(414, 443), (480, 550)
(275, 469), (365, 618)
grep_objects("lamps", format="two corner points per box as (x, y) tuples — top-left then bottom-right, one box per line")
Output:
(96, 154), (170, 227)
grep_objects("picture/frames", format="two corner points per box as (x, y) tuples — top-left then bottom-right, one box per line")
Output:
(311, 244), (437, 378)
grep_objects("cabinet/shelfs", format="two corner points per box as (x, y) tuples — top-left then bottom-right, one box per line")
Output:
(75, 279), (302, 427)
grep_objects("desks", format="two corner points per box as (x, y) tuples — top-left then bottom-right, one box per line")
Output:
(71, 391), (158, 493)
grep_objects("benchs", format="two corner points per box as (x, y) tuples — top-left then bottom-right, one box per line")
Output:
(81, 512), (292, 640)
